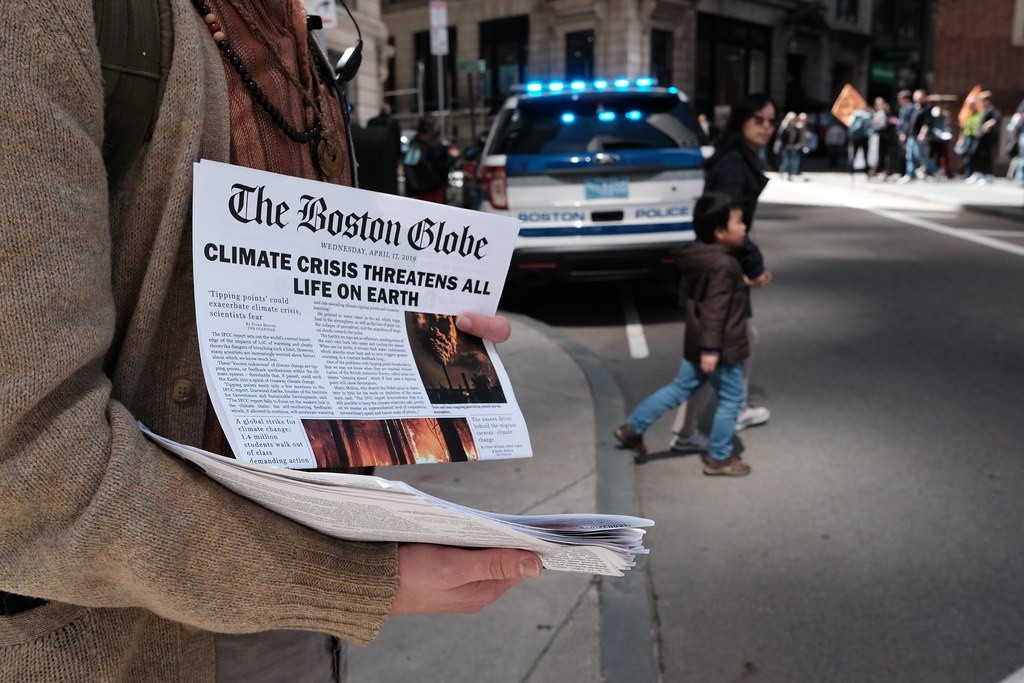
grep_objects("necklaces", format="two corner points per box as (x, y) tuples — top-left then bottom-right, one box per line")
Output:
(192, 1), (344, 176)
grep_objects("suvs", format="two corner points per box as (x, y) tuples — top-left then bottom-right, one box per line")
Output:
(469, 76), (714, 288)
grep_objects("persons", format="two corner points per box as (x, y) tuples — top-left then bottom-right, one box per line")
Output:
(610, 191), (772, 474)
(670, 92), (780, 452)
(824, 86), (1024, 184)
(0, 0), (542, 683)
(404, 115), (454, 204)
(367, 103), (400, 167)
(696, 104), (817, 179)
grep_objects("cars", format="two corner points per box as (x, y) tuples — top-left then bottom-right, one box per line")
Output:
(398, 129), (460, 168)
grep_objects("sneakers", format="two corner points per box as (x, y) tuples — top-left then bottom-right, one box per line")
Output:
(735, 407), (770, 431)
(614, 424), (647, 455)
(669, 427), (710, 451)
(704, 454), (751, 476)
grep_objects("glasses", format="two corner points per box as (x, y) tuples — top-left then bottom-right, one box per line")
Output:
(752, 114), (775, 125)
